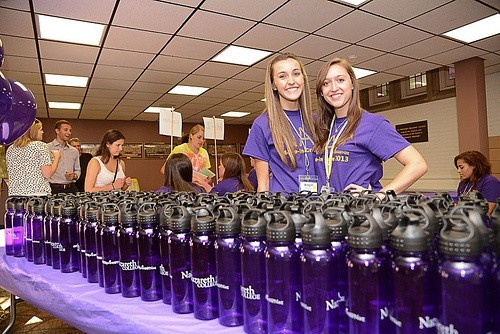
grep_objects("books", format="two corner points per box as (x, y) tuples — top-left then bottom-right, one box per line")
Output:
(198, 167), (215, 179)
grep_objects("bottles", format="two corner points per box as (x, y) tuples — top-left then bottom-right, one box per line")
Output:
(4, 190), (500, 334)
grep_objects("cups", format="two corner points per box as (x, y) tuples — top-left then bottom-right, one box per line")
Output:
(53, 150), (59, 162)
(65, 172), (72, 182)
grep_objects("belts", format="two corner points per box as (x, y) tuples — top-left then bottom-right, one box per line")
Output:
(52, 182), (75, 189)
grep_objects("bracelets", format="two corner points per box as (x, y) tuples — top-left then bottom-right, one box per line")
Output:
(112, 183), (116, 190)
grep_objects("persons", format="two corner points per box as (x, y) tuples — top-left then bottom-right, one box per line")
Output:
(153, 153), (207, 194)
(46, 120), (81, 195)
(68, 137), (93, 193)
(83, 129), (133, 193)
(5, 118), (61, 198)
(248, 155), (258, 193)
(208, 152), (254, 196)
(454, 150), (500, 216)
(160, 125), (211, 193)
(242, 53), (321, 196)
(315, 57), (429, 196)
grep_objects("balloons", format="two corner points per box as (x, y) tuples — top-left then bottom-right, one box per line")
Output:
(0, 38), (38, 147)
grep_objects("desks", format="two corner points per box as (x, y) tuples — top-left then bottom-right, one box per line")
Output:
(0, 241), (245, 334)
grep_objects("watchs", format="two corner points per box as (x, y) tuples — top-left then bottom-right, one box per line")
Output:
(72, 173), (78, 180)
